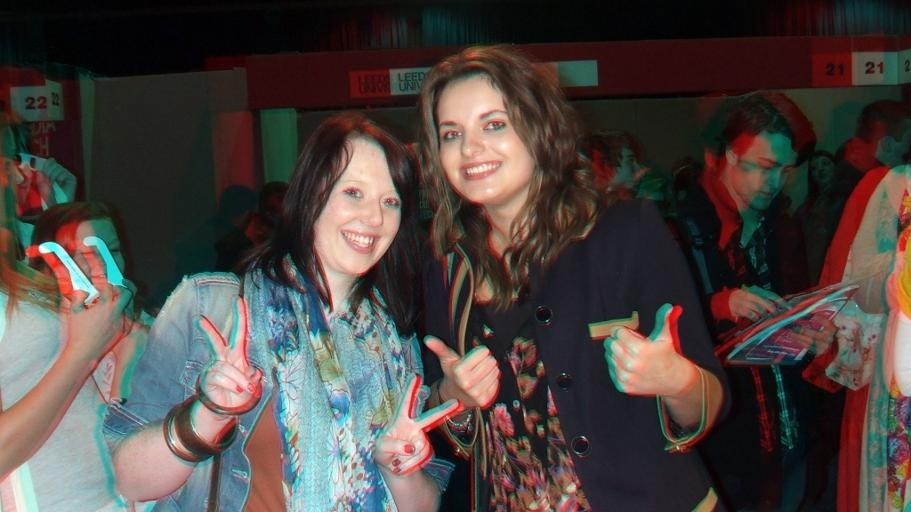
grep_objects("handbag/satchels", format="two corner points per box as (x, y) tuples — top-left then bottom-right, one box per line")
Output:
(194, 367), (264, 416)
(174, 393), (240, 458)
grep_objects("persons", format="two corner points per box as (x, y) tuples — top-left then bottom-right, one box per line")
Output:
(98, 108), (462, 512)
(216, 182), (289, 272)
(410, 43), (742, 511)
(576, 90), (909, 509)
(23, 193), (176, 424)
(0, 104), (156, 511)
(162, 406), (213, 462)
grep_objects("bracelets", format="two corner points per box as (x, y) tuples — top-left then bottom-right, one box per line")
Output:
(194, 367), (264, 416)
(446, 414), (473, 433)
(174, 393), (240, 458)
